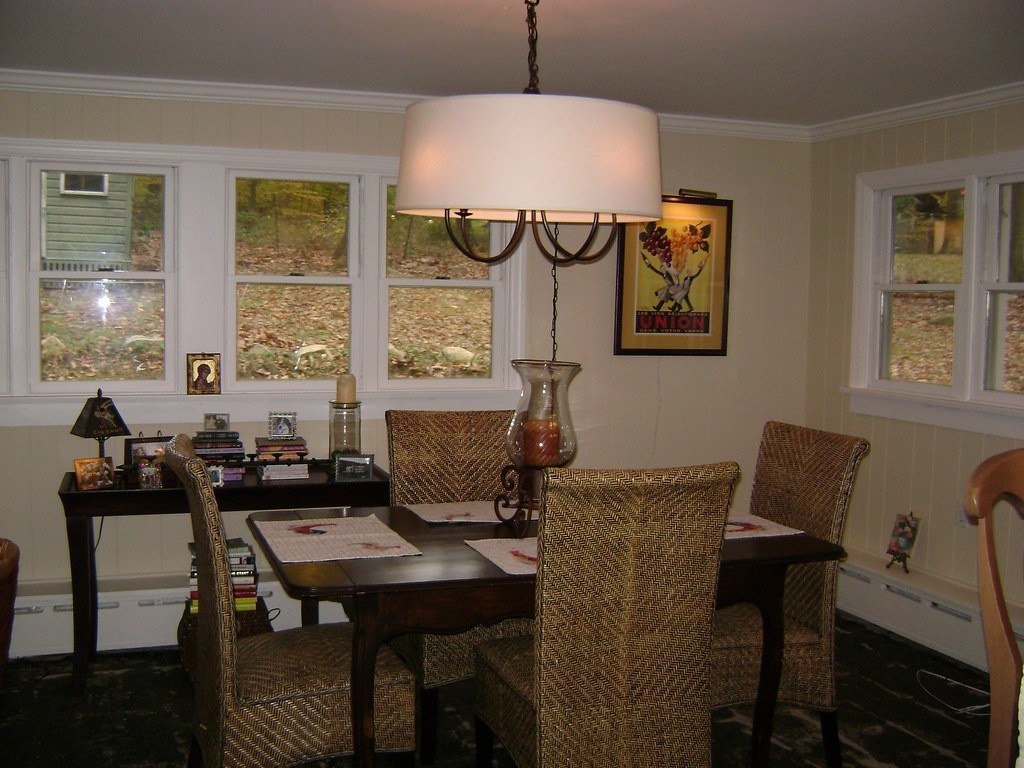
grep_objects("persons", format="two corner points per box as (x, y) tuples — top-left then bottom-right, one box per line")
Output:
(275, 418), (292, 434)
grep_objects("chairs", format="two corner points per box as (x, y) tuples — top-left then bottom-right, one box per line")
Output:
(471, 457), (745, 763)
(384, 407), (536, 694)
(165, 430), (417, 763)
(713, 417), (874, 765)
(963, 448), (1024, 767)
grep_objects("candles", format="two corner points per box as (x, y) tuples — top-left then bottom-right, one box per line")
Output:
(336, 372), (357, 402)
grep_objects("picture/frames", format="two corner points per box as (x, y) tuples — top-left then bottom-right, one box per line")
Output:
(332, 449), (377, 489)
(124, 431), (176, 469)
(184, 351), (225, 399)
(137, 461), (165, 493)
(266, 411), (304, 440)
(614, 189), (735, 357)
(202, 410), (233, 433)
(73, 459), (120, 495)
(203, 465), (225, 491)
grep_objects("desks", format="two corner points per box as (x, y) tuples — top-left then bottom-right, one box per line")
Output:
(56, 450), (393, 659)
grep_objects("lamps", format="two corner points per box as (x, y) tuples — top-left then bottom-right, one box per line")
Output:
(395, 1), (665, 267)
(69, 384), (131, 455)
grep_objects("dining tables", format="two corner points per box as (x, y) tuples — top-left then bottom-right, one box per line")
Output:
(242, 504), (851, 766)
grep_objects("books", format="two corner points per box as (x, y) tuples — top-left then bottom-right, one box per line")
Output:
(190, 429), (245, 482)
(254, 436), (310, 480)
(187, 537), (259, 614)
